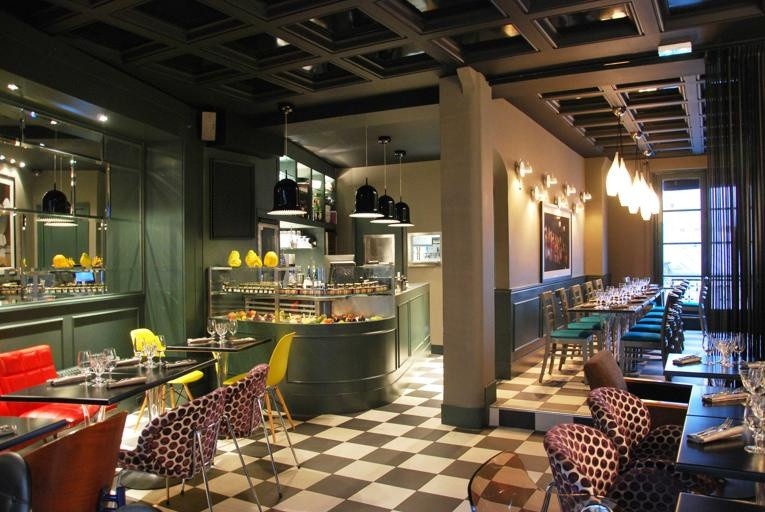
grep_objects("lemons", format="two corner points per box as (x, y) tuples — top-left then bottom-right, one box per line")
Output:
(53, 254), (66, 266)
(80, 253), (91, 265)
(264, 251), (278, 267)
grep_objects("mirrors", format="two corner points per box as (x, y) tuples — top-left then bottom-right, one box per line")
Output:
(0, 94), (147, 309)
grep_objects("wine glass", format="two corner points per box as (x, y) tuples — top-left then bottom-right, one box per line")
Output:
(77, 347), (117, 389)
(207, 317), (239, 344)
(132, 334), (166, 370)
(701, 332), (746, 368)
(737, 361), (765, 456)
(592, 275), (650, 310)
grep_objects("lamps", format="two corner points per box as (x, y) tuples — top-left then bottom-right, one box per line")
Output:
(347, 123), (387, 219)
(606, 103), (663, 223)
(265, 98), (309, 219)
(514, 157), (593, 216)
(40, 199), (79, 230)
(34, 124), (71, 222)
(385, 150), (417, 230)
(368, 133), (402, 225)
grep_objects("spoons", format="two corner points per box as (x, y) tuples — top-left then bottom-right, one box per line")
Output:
(704, 386), (745, 398)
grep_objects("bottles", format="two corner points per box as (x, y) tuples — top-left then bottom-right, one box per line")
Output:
(222, 280), (389, 296)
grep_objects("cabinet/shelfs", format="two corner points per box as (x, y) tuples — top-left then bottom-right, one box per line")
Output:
(23, 259), (106, 300)
(206, 264), (401, 420)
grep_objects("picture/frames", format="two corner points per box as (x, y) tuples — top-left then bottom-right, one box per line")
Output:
(0, 173), (20, 278)
(539, 200), (576, 285)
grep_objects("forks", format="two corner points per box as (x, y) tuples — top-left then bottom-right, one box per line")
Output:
(695, 416), (734, 439)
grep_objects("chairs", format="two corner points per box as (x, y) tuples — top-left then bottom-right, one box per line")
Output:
(537, 272), (690, 385)
(467, 354), (764, 512)
(0, 328), (302, 512)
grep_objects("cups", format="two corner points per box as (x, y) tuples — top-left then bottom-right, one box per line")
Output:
(710, 378), (726, 393)
(295, 274), (303, 285)
